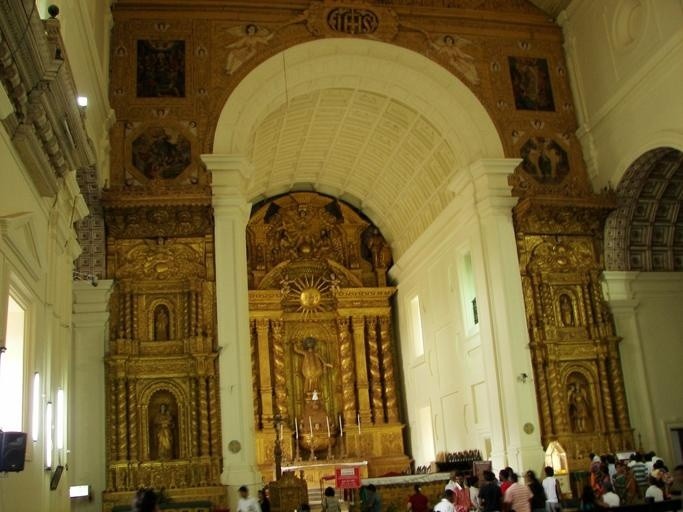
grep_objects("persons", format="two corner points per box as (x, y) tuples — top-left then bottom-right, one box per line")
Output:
(577, 451), (683, 510)
(427, 35), (479, 85)
(432, 466), (562, 512)
(562, 297), (573, 326)
(360, 483), (381, 512)
(223, 22), (269, 75)
(236, 486), (263, 512)
(257, 490), (270, 512)
(153, 403), (174, 461)
(155, 309), (169, 341)
(278, 209), (391, 296)
(572, 381), (593, 432)
(320, 487), (341, 512)
(406, 484), (431, 512)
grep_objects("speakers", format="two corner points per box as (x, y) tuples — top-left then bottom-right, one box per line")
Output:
(0, 432), (27, 472)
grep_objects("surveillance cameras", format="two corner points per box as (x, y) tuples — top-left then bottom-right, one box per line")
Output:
(91, 275), (98, 287)
